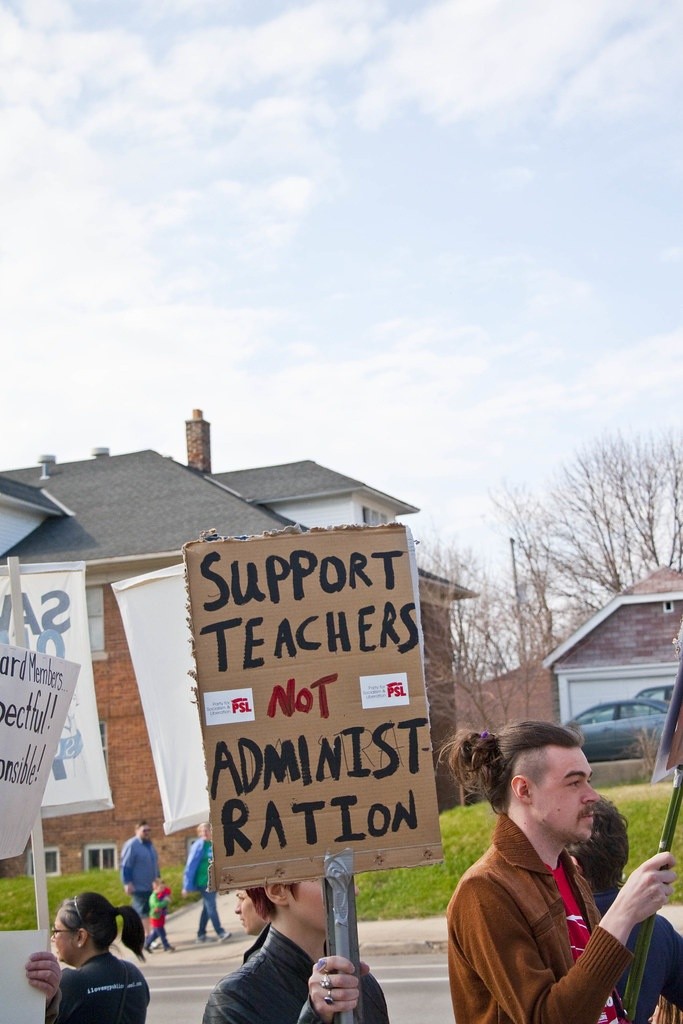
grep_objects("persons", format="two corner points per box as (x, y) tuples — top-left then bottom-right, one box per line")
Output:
(24, 952), (62, 1024)
(143, 878), (176, 954)
(202, 878), (390, 1024)
(50, 892), (150, 1024)
(181, 822), (232, 944)
(569, 794), (683, 1024)
(235, 887), (275, 966)
(437, 720), (677, 1024)
(120, 820), (161, 949)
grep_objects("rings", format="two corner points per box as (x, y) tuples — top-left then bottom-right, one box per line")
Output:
(320, 973), (332, 989)
(324, 989), (333, 1005)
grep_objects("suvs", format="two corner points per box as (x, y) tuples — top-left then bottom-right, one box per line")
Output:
(630, 685), (674, 705)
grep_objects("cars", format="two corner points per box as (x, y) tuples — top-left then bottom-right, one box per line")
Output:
(562, 698), (679, 764)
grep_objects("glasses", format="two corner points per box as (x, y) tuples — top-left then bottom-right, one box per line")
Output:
(52, 927), (95, 937)
(143, 829), (151, 833)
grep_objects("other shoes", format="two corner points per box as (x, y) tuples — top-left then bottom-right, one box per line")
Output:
(197, 934), (214, 943)
(150, 941), (161, 949)
(144, 945), (152, 953)
(219, 932), (231, 941)
(163, 947), (175, 951)
